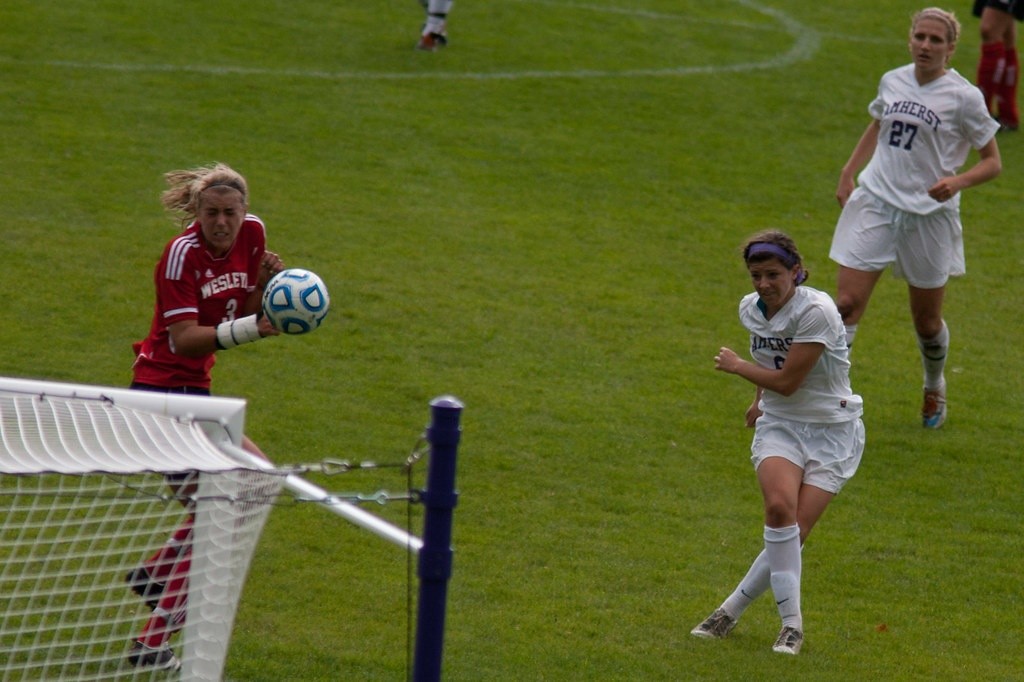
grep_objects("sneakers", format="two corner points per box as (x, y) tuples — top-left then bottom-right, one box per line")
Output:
(921, 374), (948, 429)
(772, 624), (804, 656)
(129, 639), (182, 671)
(126, 568), (165, 609)
(690, 606), (737, 640)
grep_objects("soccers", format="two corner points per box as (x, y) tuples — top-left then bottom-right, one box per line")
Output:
(261, 268), (330, 335)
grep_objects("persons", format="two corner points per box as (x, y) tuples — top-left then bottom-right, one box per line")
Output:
(124, 164), (284, 671)
(972, 0), (1024, 133)
(830, 9), (1002, 430)
(417, 0), (451, 50)
(691, 233), (866, 654)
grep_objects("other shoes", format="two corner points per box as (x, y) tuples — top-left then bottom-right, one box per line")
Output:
(994, 117), (1018, 132)
(419, 33), (446, 52)
